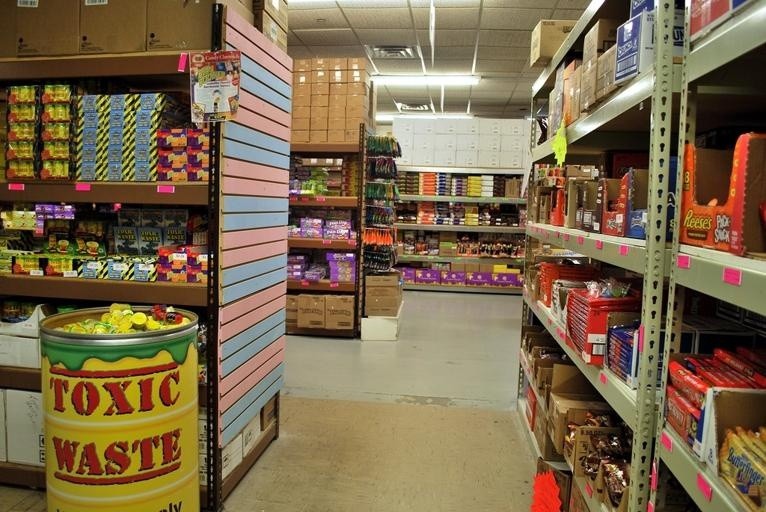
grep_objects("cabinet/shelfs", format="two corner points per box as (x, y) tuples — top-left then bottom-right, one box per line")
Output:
(397, 166), (524, 295)
(1, 2), (294, 512)
(287, 123), (403, 340)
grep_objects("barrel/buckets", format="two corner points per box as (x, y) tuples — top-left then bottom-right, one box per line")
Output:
(37, 305), (201, 510)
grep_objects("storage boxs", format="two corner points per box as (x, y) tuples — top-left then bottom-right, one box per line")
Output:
(2, 1), (288, 58)
(391, 118), (528, 167)
(516, 1), (765, 512)
(291, 58), (377, 144)
